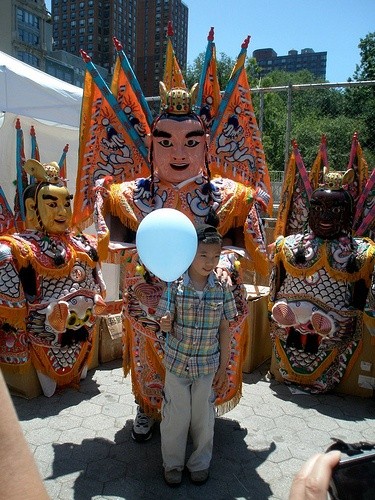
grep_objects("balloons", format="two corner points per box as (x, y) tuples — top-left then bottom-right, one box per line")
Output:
(135, 209), (197, 350)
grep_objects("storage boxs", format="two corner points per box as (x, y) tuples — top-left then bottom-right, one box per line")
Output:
(243, 284), (272, 372)
(99, 317), (124, 362)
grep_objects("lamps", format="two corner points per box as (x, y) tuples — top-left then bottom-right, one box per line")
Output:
(45, 11), (55, 26)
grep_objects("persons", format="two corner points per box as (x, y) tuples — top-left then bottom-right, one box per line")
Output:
(154, 223), (239, 484)
(94, 73), (272, 444)
(0, 372), (50, 500)
(265, 169), (375, 397)
(0, 159), (106, 399)
(287, 450), (342, 500)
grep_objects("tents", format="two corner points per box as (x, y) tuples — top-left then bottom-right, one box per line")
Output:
(0, 50), (84, 215)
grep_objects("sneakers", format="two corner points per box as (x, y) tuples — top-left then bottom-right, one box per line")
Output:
(132, 399), (156, 442)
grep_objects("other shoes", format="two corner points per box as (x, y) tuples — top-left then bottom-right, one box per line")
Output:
(162, 466), (185, 487)
(190, 468), (209, 486)
